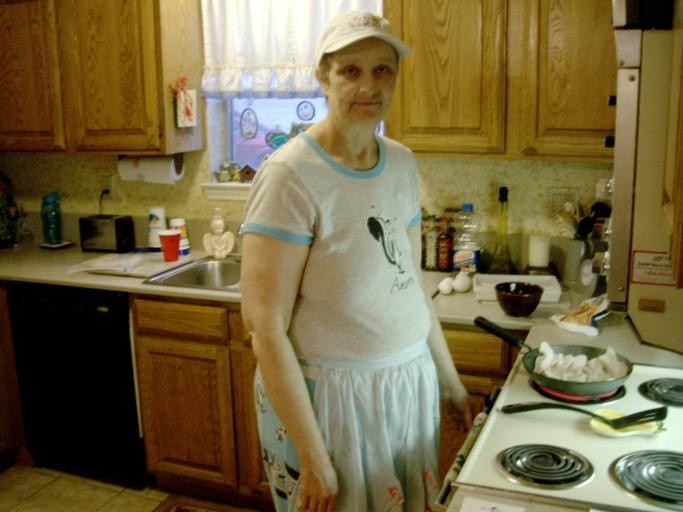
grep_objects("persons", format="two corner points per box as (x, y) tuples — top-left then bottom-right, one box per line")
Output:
(237, 11), (481, 512)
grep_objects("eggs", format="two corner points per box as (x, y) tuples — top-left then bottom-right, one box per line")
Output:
(438, 272), (471, 294)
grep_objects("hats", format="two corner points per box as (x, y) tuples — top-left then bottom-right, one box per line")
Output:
(315, 8), (417, 70)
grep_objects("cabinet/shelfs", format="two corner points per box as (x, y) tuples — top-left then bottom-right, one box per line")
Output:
(128, 296), (273, 509)
(0, 2), (209, 157)
(379, 0), (616, 165)
(439, 329), (528, 503)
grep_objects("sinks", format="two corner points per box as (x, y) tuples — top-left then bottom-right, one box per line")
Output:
(143, 256), (242, 292)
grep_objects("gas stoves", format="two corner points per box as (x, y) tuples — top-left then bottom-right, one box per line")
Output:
(450, 351), (683, 512)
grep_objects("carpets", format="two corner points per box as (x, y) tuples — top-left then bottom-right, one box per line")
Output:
(151, 493), (256, 512)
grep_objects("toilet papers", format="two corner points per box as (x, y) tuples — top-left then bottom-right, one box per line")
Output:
(117, 158), (183, 185)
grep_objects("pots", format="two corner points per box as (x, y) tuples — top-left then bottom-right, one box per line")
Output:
(474, 316), (633, 396)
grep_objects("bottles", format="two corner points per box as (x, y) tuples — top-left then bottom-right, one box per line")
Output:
(422, 186), (516, 273)
(41, 194), (64, 244)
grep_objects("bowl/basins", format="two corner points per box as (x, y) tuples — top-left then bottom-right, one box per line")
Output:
(494, 281), (543, 315)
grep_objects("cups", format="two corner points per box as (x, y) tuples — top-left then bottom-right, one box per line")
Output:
(148, 207), (190, 262)
(527, 230), (551, 268)
